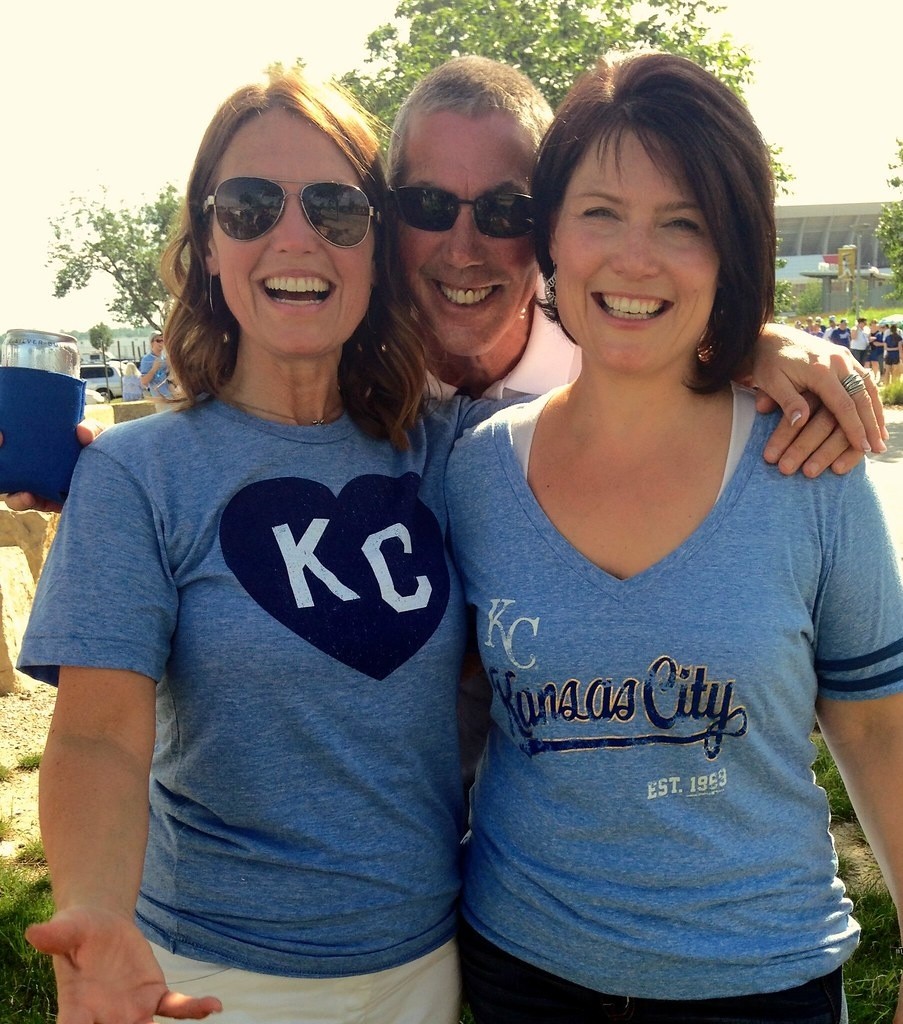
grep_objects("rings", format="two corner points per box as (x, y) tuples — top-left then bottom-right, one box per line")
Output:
(863, 372), (869, 379)
(843, 371), (866, 396)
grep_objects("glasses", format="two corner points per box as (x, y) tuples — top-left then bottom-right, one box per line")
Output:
(203, 176), (381, 248)
(388, 184), (537, 238)
(152, 339), (163, 343)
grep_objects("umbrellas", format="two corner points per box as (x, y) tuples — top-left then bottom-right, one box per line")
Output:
(880, 314), (902, 327)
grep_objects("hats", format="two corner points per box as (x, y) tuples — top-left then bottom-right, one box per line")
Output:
(829, 315), (836, 320)
(841, 318), (849, 323)
(149, 330), (163, 342)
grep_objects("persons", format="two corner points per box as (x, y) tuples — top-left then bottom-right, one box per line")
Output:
(446, 57), (903, 1023)
(18, 74), (889, 1024)
(121, 362), (144, 401)
(6, 48), (864, 1024)
(140, 331), (185, 413)
(794, 315), (903, 386)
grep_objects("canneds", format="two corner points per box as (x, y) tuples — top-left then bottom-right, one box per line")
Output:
(0, 329), (87, 501)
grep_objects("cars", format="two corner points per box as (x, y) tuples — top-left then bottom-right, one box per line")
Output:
(80, 366), (124, 401)
